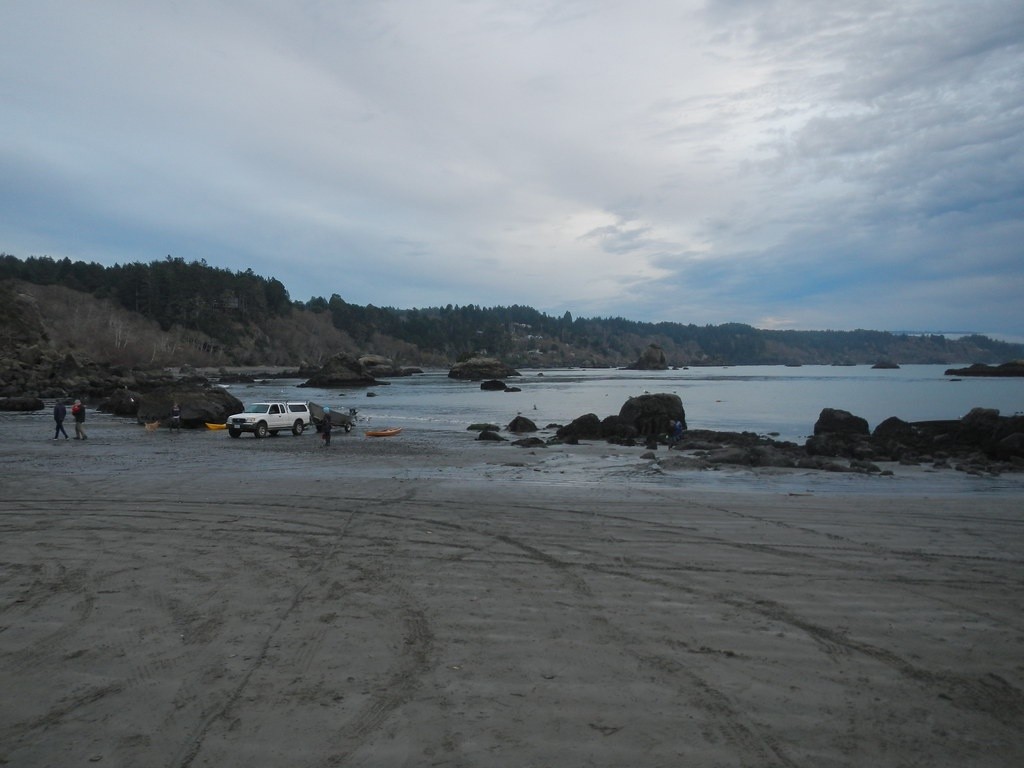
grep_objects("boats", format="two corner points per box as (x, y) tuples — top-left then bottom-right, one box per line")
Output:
(364, 426), (403, 437)
(205, 421), (227, 431)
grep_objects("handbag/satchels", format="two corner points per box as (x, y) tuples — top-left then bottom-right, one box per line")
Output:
(322, 433), (325, 440)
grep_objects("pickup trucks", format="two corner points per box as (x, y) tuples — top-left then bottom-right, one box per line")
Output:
(227, 401), (311, 438)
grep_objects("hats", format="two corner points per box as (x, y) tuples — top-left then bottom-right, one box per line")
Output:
(323, 407), (329, 414)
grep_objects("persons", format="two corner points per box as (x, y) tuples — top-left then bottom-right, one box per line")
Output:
(53, 399), (70, 441)
(169, 402), (182, 434)
(666, 420), (683, 451)
(321, 412), (332, 446)
(72, 400), (89, 440)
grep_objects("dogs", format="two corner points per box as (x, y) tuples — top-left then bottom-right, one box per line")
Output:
(145, 420), (161, 432)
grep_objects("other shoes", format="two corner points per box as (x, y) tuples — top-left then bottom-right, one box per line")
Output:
(65, 438), (70, 441)
(53, 439), (58, 441)
(81, 435), (87, 440)
(74, 437), (80, 440)
